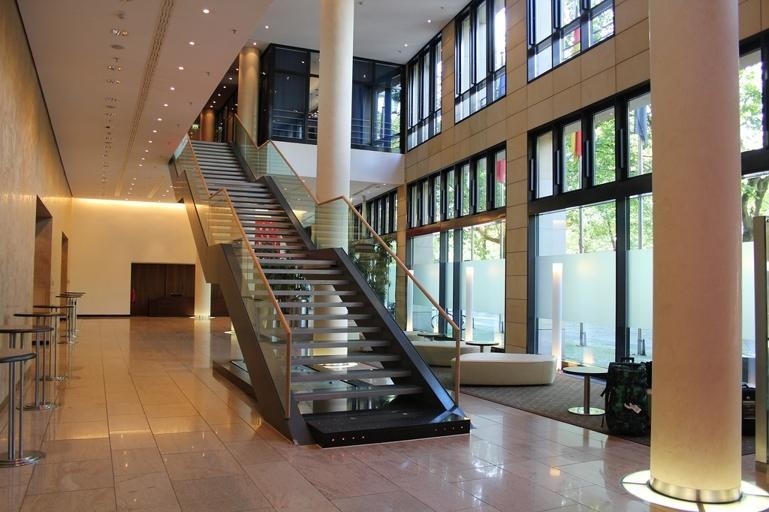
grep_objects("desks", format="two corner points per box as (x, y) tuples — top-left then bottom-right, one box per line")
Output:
(419, 333), (447, 342)
(465, 341), (500, 354)
(561, 365), (607, 416)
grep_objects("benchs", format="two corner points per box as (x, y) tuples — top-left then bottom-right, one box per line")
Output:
(451, 351), (557, 385)
(409, 339), (487, 366)
(403, 330), (424, 341)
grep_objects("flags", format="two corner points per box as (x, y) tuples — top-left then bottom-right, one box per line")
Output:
(634, 106), (647, 142)
(496, 74), (506, 184)
(570, 27), (582, 156)
